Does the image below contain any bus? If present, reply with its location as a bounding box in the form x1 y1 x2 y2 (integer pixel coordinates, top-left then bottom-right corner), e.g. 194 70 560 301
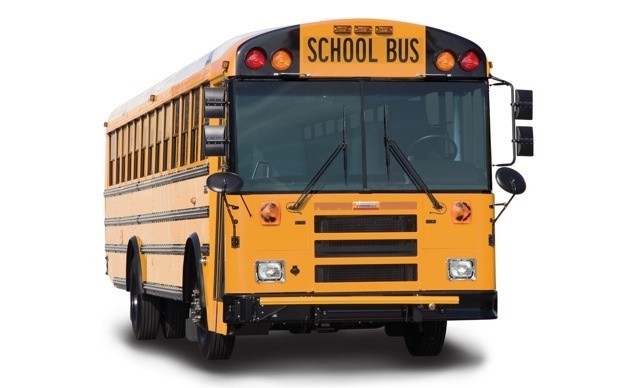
104 20 534 358
